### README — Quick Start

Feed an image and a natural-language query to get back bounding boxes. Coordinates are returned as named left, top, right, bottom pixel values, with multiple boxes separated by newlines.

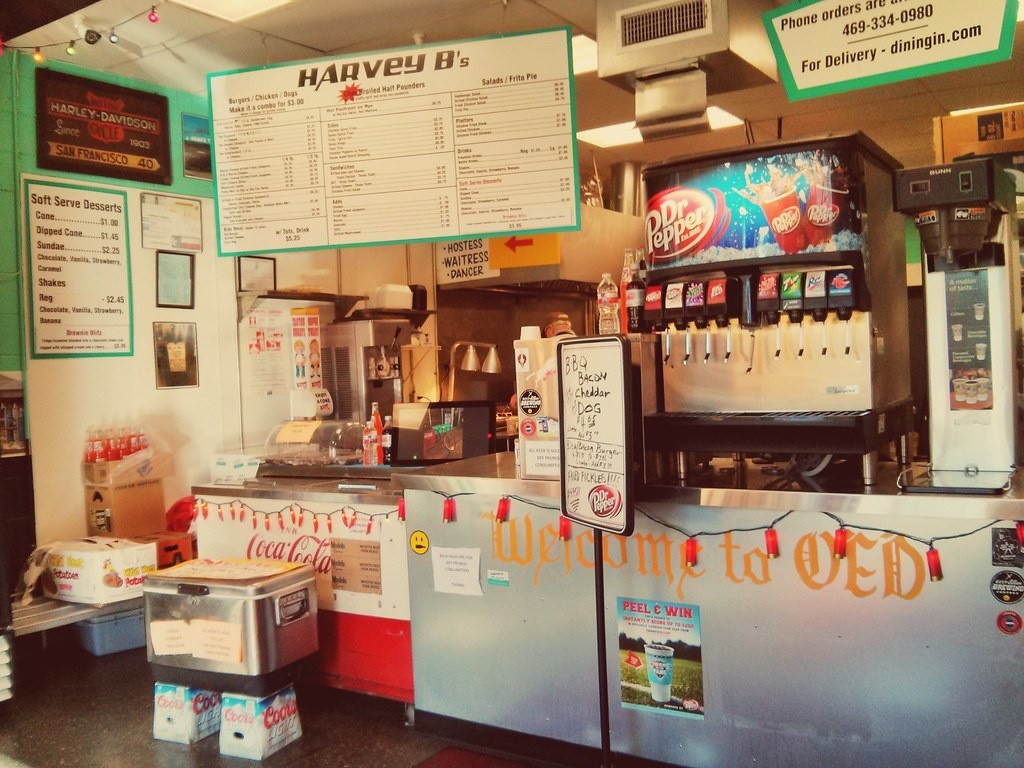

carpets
left=412, top=744, right=544, bottom=768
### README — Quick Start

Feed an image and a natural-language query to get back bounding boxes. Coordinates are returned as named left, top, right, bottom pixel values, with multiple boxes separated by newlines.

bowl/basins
left=520, top=327, right=540, bottom=340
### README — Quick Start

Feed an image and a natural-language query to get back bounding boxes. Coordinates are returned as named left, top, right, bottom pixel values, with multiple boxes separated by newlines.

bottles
left=362, top=420, right=378, bottom=466
left=381, top=416, right=396, bottom=465
left=84, top=427, right=148, bottom=462
left=370, top=402, right=384, bottom=465
left=618, top=245, right=647, bottom=332
left=597, top=273, right=620, bottom=335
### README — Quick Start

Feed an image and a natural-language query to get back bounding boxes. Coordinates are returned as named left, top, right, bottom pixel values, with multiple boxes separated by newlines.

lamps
left=447, top=339, right=504, bottom=401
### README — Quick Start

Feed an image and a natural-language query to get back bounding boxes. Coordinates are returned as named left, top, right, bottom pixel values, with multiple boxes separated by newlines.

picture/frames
left=155, top=250, right=196, bottom=310
left=238, top=254, right=277, bottom=293
left=152, top=321, right=200, bottom=390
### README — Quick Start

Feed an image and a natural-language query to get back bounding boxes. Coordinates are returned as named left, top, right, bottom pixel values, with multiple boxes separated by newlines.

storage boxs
left=941, top=103, right=1024, bottom=165
left=39, top=449, right=322, bottom=761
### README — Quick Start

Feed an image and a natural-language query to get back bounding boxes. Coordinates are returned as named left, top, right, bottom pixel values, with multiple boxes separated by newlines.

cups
left=644, top=644, right=674, bottom=703
left=952, top=324, right=963, bottom=341
left=976, top=343, right=987, bottom=361
left=952, top=379, right=966, bottom=401
left=964, top=380, right=978, bottom=404
left=976, top=377, right=989, bottom=401
left=760, top=186, right=805, bottom=255
left=974, top=302, right=985, bottom=320
left=804, top=184, right=848, bottom=246
